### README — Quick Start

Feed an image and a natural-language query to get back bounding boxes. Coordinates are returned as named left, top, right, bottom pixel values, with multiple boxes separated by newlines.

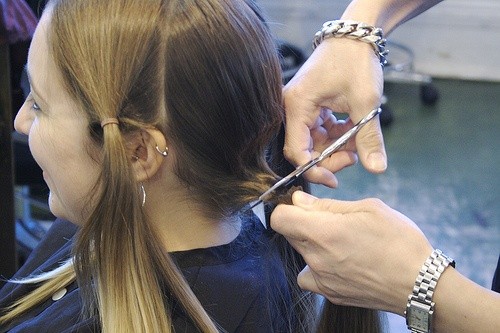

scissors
left=236, top=105, right=382, bottom=216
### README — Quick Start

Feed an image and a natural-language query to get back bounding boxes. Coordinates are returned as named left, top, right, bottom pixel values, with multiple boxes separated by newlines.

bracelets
left=311, top=20, right=389, bottom=66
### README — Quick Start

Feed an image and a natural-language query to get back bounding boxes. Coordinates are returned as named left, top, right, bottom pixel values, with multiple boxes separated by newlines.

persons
left=0, top=0, right=316, bottom=333
left=269, top=1, right=500, bottom=332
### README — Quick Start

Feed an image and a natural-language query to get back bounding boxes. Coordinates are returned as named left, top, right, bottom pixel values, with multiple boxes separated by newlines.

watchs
left=404, top=247, right=457, bottom=333
